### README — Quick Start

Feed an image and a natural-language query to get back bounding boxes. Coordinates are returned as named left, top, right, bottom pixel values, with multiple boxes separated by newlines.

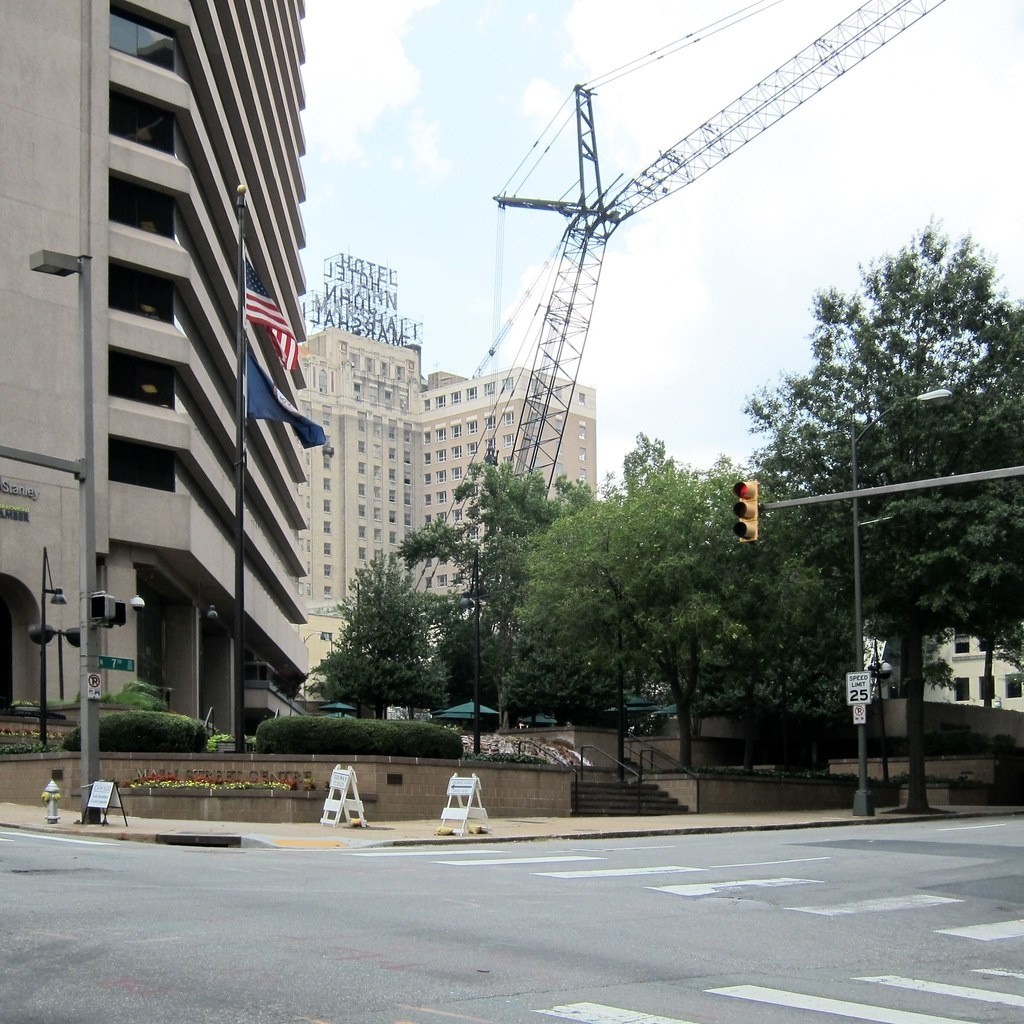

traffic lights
left=732, top=481, right=759, bottom=541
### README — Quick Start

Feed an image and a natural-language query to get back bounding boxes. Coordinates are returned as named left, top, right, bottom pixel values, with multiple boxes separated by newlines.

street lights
left=851, top=389, right=953, bottom=816
left=868, top=636, right=893, bottom=784
left=39, top=547, right=67, bottom=744
left=459, top=552, right=490, bottom=754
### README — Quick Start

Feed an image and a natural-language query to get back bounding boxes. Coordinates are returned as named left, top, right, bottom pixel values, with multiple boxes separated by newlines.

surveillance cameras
left=131, top=596, right=145, bottom=612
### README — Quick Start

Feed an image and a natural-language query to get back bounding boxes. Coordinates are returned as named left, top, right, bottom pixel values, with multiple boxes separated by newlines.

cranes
left=415, top=0, right=946, bottom=594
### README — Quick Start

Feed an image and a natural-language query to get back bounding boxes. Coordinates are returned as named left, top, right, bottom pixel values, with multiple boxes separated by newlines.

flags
left=247, top=352, right=327, bottom=449
left=242, top=239, right=299, bottom=372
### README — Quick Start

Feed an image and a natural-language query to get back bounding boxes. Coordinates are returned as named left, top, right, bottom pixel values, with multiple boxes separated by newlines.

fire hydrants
left=41, top=778, right=61, bottom=824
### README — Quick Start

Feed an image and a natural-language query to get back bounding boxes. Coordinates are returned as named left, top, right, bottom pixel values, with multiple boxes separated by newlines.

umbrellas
left=605, top=696, right=695, bottom=716
left=430, top=700, right=499, bottom=732
left=522, top=714, right=558, bottom=724
left=318, top=702, right=356, bottom=718
